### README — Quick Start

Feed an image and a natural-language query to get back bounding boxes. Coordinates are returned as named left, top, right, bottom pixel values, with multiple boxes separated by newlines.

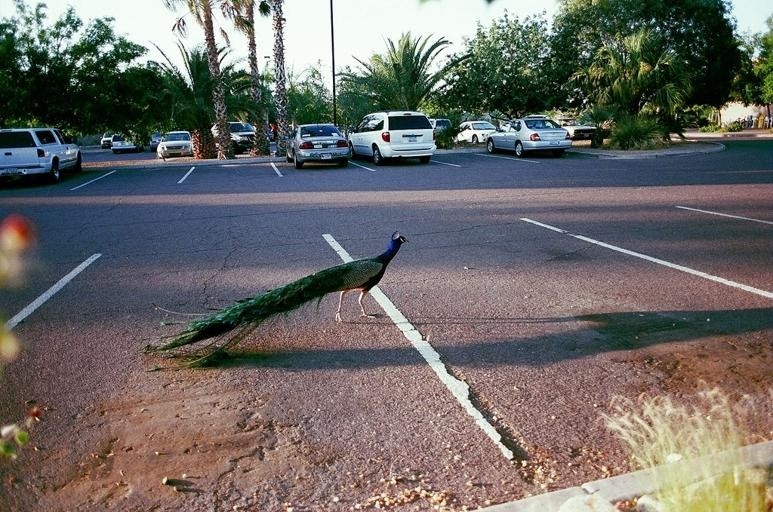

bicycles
left=732, top=116, right=749, bottom=131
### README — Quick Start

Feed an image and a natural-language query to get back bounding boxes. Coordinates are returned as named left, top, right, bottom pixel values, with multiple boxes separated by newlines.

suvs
left=211, top=121, right=256, bottom=155
left=428, top=118, right=453, bottom=138
left=347, top=110, right=437, bottom=166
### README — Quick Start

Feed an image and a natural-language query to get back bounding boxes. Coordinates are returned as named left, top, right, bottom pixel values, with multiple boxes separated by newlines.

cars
left=99, top=129, right=193, bottom=159
left=522, top=114, right=550, bottom=127
left=452, top=120, right=497, bottom=145
left=486, top=118, right=573, bottom=159
left=284, top=124, right=350, bottom=169
left=0, top=127, right=82, bottom=182
left=545, top=117, right=598, bottom=142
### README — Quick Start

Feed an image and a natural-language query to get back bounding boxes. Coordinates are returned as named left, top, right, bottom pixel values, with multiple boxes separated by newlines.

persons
left=270, top=122, right=278, bottom=139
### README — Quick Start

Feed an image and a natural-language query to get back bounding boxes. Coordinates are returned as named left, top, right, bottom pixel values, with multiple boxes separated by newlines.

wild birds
left=140, top=230, right=410, bottom=372
